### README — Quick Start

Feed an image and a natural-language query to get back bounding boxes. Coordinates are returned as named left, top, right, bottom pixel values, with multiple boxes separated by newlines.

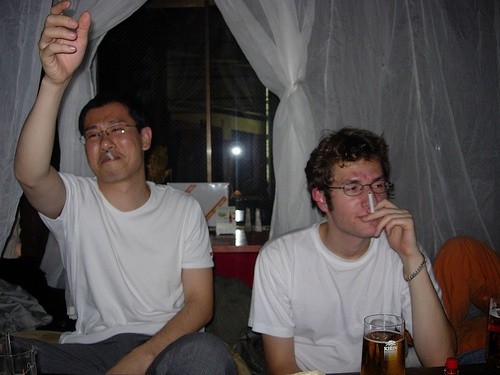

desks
left=211, top=230, right=269, bottom=287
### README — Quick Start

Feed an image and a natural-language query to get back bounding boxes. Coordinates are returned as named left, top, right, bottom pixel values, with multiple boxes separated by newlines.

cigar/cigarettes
left=368, top=191, right=374, bottom=214
left=105, top=149, right=115, bottom=161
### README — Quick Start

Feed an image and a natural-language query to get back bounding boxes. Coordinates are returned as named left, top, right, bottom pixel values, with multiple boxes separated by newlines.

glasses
left=78, top=123, right=135, bottom=143
left=328, top=182, right=386, bottom=197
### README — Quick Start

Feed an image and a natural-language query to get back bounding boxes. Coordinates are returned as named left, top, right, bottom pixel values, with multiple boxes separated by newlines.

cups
left=0, top=341, right=37, bottom=375
left=360, top=314, right=405, bottom=375
left=482, top=297, right=500, bottom=375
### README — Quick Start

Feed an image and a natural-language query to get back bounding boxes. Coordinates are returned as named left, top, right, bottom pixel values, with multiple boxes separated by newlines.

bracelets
left=405, top=253, right=427, bottom=283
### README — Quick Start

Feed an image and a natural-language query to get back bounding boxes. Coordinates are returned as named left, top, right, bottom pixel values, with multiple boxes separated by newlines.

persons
left=13, top=0, right=238, bottom=375
left=433, top=235, right=500, bottom=355
left=248, top=126, right=458, bottom=375
